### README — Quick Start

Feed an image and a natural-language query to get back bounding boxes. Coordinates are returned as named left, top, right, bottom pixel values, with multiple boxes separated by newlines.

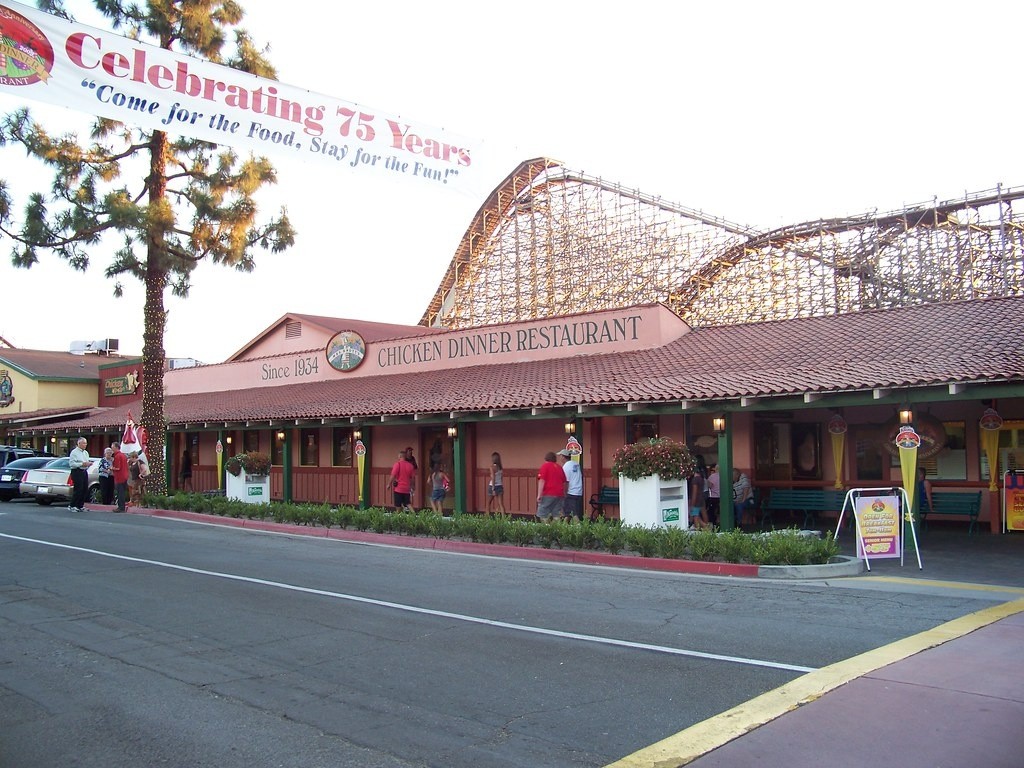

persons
left=426, top=461, right=450, bottom=518
left=917, top=467, right=935, bottom=513
left=67, top=437, right=93, bottom=512
left=688, top=455, right=754, bottom=531
left=387, top=447, right=418, bottom=514
left=98, top=442, right=147, bottom=513
left=485, top=452, right=505, bottom=520
left=537, top=449, right=582, bottom=526
left=180, top=450, right=195, bottom=494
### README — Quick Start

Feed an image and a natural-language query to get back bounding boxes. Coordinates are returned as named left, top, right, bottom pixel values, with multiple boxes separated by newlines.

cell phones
left=90, top=460, right=94, bottom=463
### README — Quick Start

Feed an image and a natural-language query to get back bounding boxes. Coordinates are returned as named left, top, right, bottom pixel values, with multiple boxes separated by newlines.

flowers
left=224, top=450, right=271, bottom=477
left=612, top=434, right=705, bottom=482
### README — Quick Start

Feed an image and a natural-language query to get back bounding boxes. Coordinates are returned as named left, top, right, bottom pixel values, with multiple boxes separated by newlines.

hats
left=555, top=449, right=572, bottom=456
left=405, top=446, right=413, bottom=451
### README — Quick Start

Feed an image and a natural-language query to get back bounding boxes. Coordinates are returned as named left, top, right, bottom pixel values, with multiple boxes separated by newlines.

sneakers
left=113, top=508, right=125, bottom=512
left=78, top=506, right=89, bottom=511
left=68, top=505, right=79, bottom=512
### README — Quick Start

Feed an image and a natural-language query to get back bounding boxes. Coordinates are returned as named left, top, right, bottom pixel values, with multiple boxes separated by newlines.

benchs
left=702, top=486, right=759, bottom=526
left=761, top=490, right=860, bottom=530
left=588, top=486, right=619, bottom=518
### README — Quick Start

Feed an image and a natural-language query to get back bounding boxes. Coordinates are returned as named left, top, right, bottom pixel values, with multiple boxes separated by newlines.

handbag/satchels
left=442, top=479, right=449, bottom=491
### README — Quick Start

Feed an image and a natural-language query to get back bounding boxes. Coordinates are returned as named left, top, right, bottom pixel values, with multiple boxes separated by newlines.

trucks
left=0, top=445, right=35, bottom=468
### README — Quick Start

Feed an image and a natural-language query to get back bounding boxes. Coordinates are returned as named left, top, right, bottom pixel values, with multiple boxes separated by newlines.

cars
left=20, top=456, right=105, bottom=507
left=0, top=457, right=67, bottom=503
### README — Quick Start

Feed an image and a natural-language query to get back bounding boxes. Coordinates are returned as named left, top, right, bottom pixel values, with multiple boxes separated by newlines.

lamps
left=227, top=434, right=232, bottom=445
left=277, top=431, right=286, bottom=441
left=899, top=408, right=912, bottom=426
left=565, top=421, right=575, bottom=436
left=447, top=425, right=458, bottom=439
left=51, top=436, right=56, bottom=444
left=880, top=491, right=983, bottom=537
left=353, top=429, right=361, bottom=439
left=714, top=418, right=725, bottom=432
